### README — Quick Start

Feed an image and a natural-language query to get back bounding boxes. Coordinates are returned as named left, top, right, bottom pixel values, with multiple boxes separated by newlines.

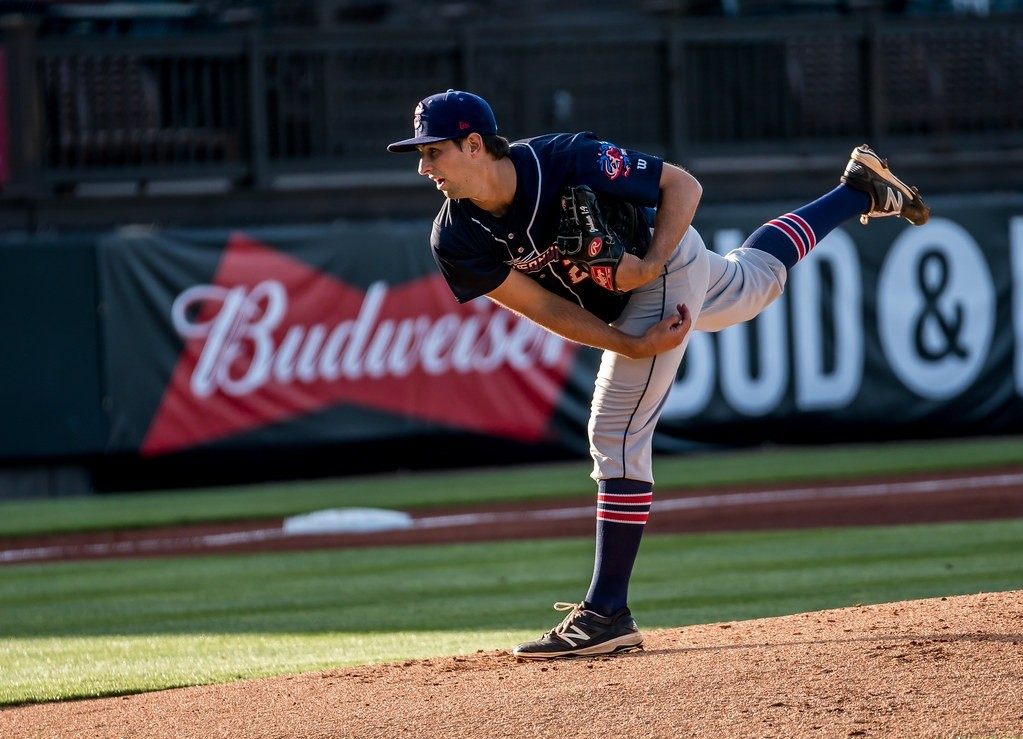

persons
left=387, top=87, right=930, bottom=661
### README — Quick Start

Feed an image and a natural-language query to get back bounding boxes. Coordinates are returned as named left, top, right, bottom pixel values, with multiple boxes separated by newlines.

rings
left=616, top=285, right=620, bottom=291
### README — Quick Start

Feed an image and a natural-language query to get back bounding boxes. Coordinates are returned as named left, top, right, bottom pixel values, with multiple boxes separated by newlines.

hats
left=387, top=88, right=496, bottom=153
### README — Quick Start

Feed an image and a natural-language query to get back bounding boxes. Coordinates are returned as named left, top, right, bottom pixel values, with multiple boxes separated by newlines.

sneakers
left=512, top=600, right=643, bottom=661
left=841, top=143, right=931, bottom=227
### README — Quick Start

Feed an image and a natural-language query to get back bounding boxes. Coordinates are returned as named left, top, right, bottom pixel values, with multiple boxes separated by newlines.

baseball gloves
left=554, top=182, right=639, bottom=297
